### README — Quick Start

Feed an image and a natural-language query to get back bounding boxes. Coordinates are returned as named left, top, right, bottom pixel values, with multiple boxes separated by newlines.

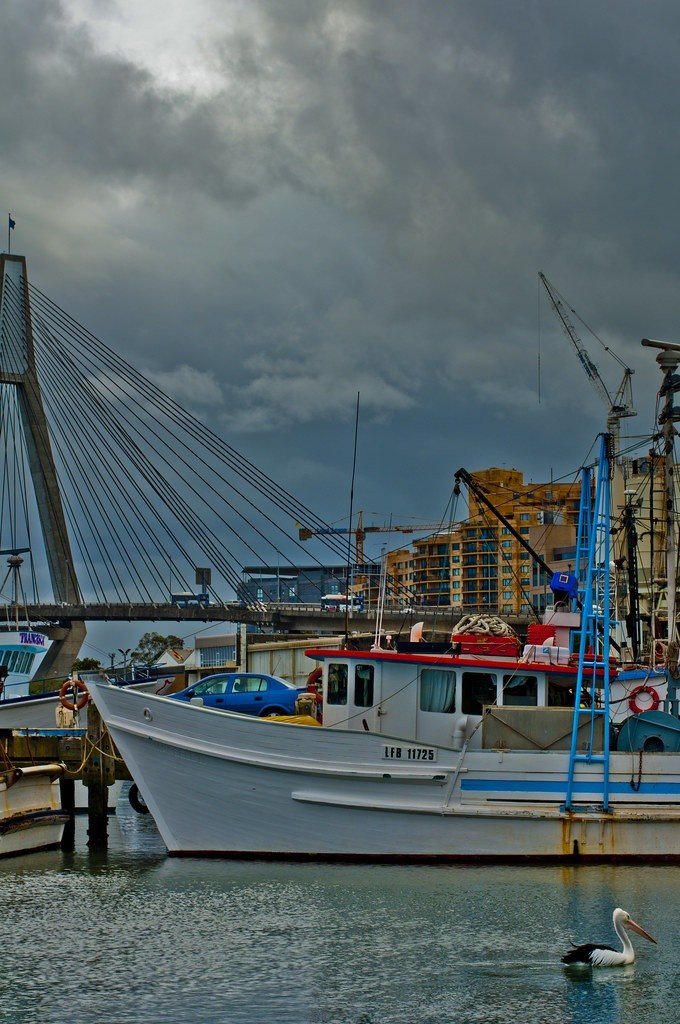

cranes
left=297, top=511, right=461, bottom=613
left=536, top=270, right=637, bottom=458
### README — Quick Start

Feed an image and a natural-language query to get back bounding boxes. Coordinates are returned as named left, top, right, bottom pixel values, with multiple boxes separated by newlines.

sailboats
left=80, top=259, right=680, bottom=855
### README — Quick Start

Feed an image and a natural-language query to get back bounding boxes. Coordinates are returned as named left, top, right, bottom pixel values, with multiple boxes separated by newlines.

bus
left=320, top=594, right=364, bottom=613
left=169, top=592, right=209, bottom=608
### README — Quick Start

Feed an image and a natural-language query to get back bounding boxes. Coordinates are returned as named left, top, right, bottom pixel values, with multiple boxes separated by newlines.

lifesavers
left=628, top=686, right=659, bottom=713
left=128, top=783, right=150, bottom=814
left=569, top=652, right=617, bottom=670
left=306, top=666, right=343, bottom=703
left=60, top=680, right=88, bottom=710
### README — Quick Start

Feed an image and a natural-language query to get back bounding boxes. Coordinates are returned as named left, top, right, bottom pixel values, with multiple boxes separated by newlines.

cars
left=55, top=601, right=71, bottom=608
left=161, top=673, right=310, bottom=717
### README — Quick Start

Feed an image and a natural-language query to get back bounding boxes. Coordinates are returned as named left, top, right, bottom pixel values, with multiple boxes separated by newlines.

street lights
left=118, top=647, right=132, bottom=683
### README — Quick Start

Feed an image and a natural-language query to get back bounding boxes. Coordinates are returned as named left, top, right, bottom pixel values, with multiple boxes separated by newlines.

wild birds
left=562, top=907, right=658, bottom=968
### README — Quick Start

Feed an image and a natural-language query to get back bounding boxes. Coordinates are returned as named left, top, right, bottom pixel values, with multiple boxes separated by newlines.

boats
left=0, top=664, right=175, bottom=815
left=0, top=759, right=70, bottom=857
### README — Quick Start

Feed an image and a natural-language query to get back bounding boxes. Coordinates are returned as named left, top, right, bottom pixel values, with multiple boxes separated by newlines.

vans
left=583, top=604, right=603, bottom=616
left=223, top=599, right=240, bottom=609
left=545, top=605, right=554, bottom=613
